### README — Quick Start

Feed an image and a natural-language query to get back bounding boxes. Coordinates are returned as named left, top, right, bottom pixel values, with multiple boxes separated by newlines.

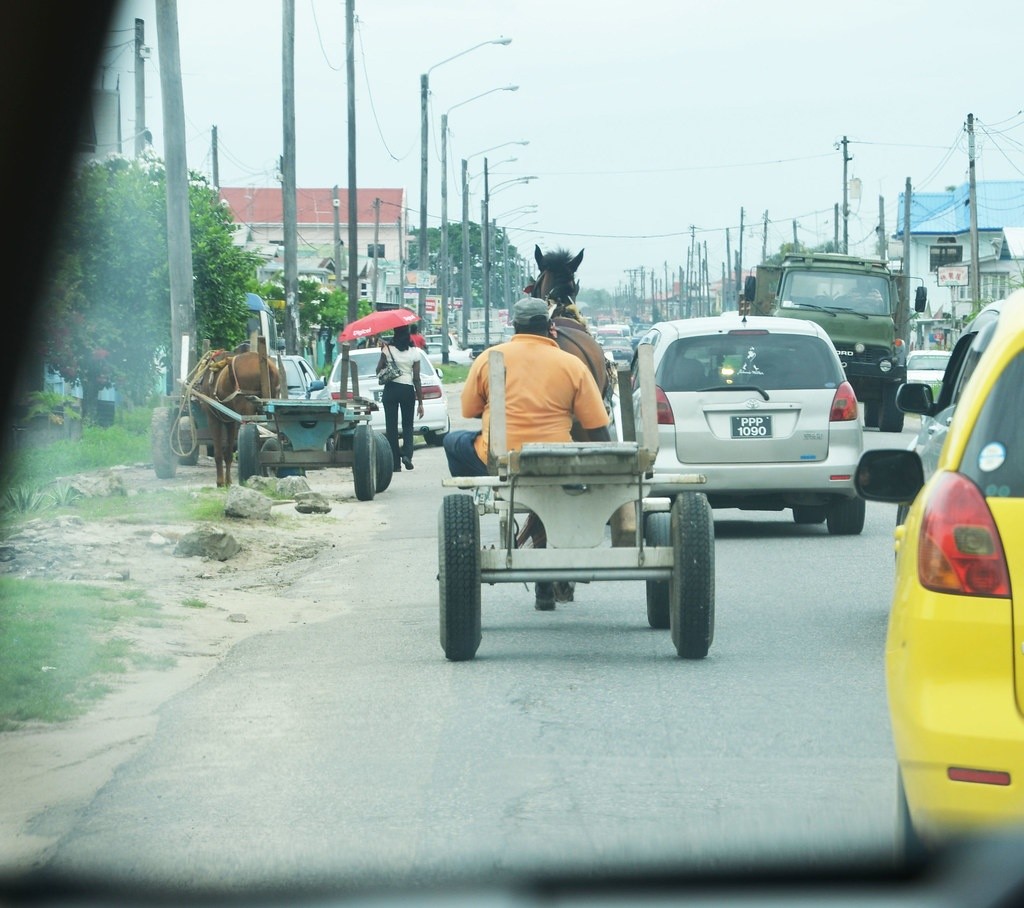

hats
left=508, top=296, right=550, bottom=326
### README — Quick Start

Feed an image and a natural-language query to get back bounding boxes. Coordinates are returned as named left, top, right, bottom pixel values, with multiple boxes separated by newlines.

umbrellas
left=339, top=307, right=421, bottom=347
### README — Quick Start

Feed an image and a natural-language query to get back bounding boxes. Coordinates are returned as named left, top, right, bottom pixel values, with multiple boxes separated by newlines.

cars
left=623, top=313, right=868, bottom=536
left=902, top=350, right=956, bottom=405
left=267, top=354, right=324, bottom=405
left=451, top=306, right=658, bottom=372
left=852, top=287, right=1024, bottom=877
left=887, top=299, right=1005, bottom=534
left=326, top=346, right=452, bottom=448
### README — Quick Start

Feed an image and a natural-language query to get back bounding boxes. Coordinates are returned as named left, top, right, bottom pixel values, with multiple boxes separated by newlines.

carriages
left=172, top=339, right=394, bottom=503
left=436, top=243, right=722, bottom=664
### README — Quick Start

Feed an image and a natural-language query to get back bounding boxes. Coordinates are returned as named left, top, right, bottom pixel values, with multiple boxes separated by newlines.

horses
left=196, top=353, right=279, bottom=488
left=517, top=244, right=615, bottom=610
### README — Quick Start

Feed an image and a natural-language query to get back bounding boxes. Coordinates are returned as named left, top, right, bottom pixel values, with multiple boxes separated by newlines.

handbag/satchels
left=378, top=342, right=402, bottom=385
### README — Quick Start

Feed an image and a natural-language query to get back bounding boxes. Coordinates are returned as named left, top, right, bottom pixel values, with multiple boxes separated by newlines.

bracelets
left=418, top=400, right=422, bottom=406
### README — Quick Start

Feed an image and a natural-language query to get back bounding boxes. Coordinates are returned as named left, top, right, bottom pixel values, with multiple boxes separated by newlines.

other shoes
left=393, top=466, right=402, bottom=472
left=402, top=456, right=414, bottom=470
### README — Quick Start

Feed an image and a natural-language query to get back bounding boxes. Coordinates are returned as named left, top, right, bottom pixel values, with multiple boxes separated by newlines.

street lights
left=439, top=84, right=519, bottom=362
left=417, top=36, right=513, bottom=360
left=460, top=139, right=530, bottom=347
left=482, top=157, right=541, bottom=342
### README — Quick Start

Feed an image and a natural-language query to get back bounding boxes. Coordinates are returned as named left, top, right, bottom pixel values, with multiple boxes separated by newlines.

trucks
left=741, top=256, right=929, bottom=431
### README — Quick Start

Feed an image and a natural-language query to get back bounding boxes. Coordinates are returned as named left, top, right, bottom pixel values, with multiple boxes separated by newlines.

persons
left=408, top=325, right=429, bottom=355
left=443, top=298, right=613, bottom=492
left=377, top=325, right=425, bottom=471
